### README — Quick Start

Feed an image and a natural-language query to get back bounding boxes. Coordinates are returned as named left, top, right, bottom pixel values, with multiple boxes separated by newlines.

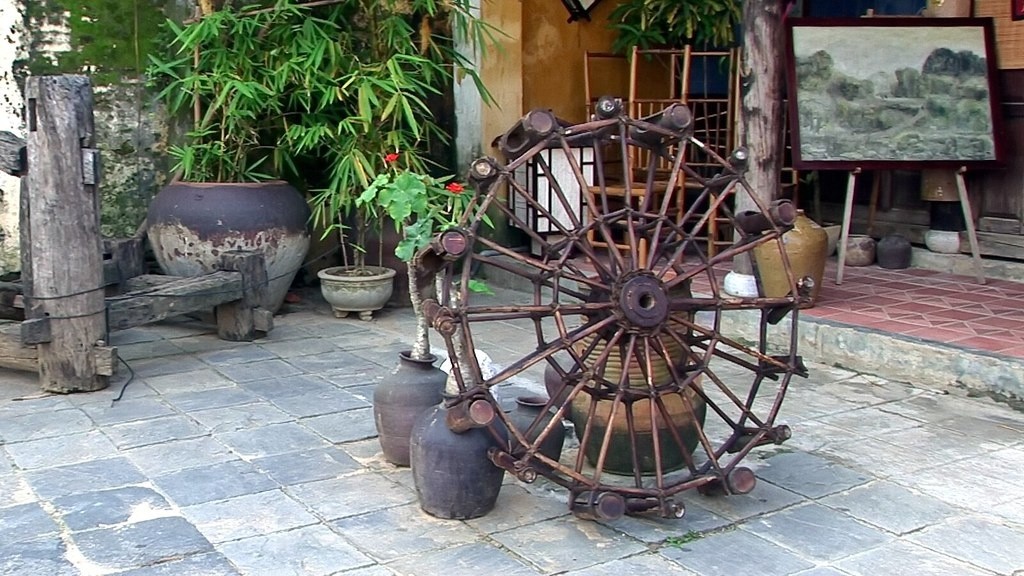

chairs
left=584, top=44, right=799, bottom=269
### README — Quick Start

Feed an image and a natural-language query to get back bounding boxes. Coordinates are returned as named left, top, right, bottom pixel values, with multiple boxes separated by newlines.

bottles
left=506, top=270, right=707, bottom=476
left=753, top=209, right=829, bottom=309
left=925, top=230, right=960, bottom=254
left=835, top=234, right=875, bottom=267
left=876, top=232, right=912, bottom=269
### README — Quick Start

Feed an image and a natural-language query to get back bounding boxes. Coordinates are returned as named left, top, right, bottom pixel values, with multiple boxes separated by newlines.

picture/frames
left=784, top=16, right=1005, bottom=170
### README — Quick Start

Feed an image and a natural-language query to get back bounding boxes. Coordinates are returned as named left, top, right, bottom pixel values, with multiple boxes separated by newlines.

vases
left=374, top=351, right=446, bottom=467
left=409, top=393, right=506, bottom=520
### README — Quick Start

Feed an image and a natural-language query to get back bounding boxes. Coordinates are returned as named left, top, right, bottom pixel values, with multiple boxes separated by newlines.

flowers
left=353, top=152, right=496, bottom=395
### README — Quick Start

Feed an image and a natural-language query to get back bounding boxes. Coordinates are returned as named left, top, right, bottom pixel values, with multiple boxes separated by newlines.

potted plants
left=318, top=206, right=404, bottom=309
left=144, top=0, right=511, bottom=321
